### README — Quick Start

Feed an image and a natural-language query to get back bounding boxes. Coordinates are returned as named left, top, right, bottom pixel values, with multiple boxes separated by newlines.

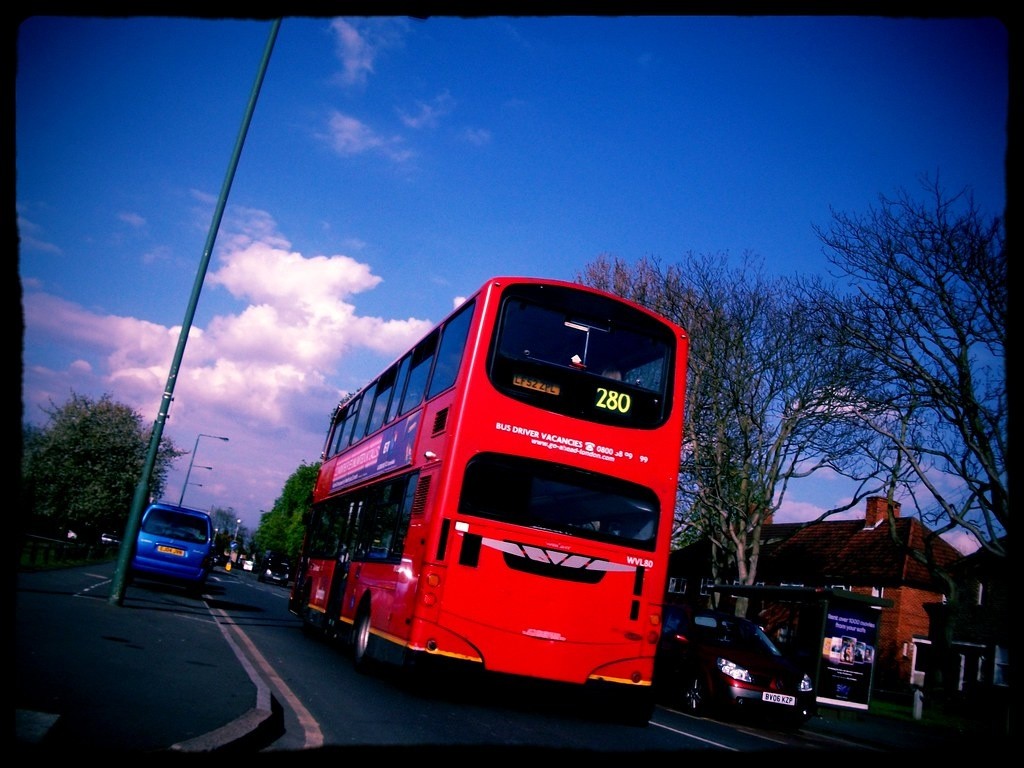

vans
left=131, top=502, right=214, bottom=598
left=257, top=551, right=292, bottom=586
left=243, top=560, right=254, bottom=573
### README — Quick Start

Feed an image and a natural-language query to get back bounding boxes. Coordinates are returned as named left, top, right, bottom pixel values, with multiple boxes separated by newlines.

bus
left=288, top=280, right=691, bottom=701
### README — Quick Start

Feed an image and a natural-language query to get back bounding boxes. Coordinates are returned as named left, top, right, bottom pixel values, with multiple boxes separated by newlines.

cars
left=651, top=602, right=815, bottom=732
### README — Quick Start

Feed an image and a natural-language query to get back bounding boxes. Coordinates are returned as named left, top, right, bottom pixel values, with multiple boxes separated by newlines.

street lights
left=178, top=433, right=229, bottom=506
